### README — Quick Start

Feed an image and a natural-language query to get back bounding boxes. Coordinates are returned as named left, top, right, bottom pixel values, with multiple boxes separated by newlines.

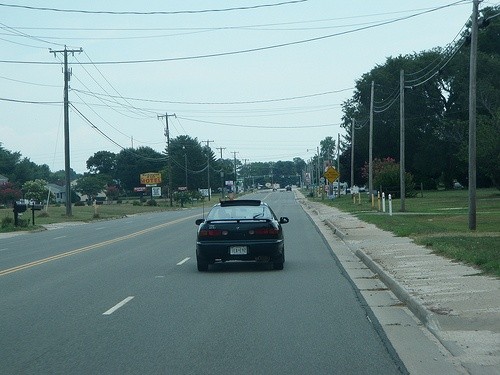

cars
left=285, top=187, right=292, bottom=191
left=273, top=187, right=277, bottom=191
left=195, top=199, right=289, bottom=273
